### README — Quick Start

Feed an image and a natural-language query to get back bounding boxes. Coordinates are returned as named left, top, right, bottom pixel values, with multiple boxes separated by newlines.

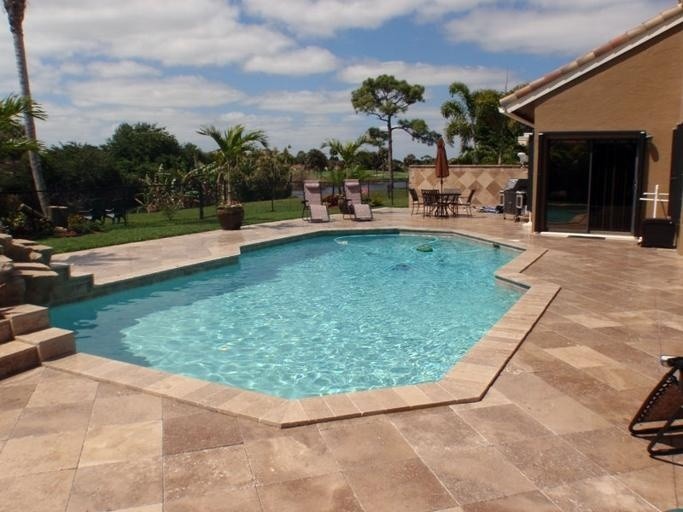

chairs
left=104, top=199, right=130, bottom=225
left=407, top=186, right=476, bottom=219
left=77, top=197, right=109, bottom=226
left=341, top=178, right=372, bottom=221
left=627, top=354, right=683, bottom=466
left=299, top=180, right=329, bottom=223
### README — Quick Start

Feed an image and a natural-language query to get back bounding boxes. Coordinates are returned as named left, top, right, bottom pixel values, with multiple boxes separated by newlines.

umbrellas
left=436, top=138, right=449, bottom=215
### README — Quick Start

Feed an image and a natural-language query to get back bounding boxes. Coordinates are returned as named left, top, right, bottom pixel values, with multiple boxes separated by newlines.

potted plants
left=196, top=125, right=270, bottom=230
left=320, top=135, right=382, bottom=211
